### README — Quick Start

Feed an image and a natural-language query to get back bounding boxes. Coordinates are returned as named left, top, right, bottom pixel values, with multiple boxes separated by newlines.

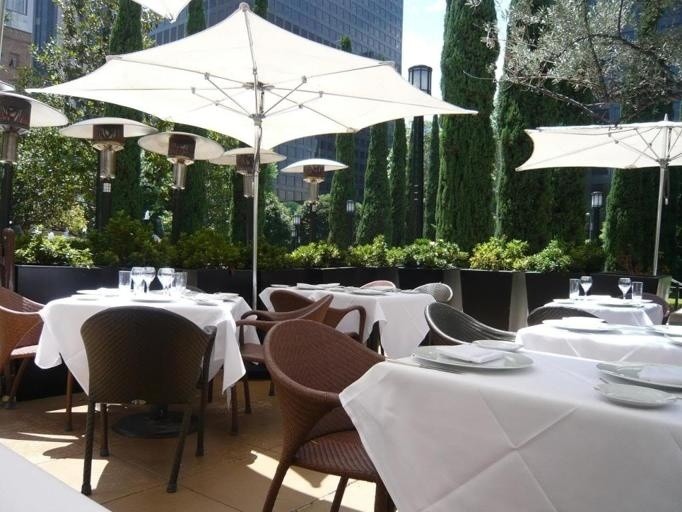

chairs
left=618, top=293, right=671, bottom=325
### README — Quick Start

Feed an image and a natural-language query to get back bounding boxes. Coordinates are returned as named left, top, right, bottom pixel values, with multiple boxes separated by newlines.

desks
left=375, top=296, right=682, bottom=512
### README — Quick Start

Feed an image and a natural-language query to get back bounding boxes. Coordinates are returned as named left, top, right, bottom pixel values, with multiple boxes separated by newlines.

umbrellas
left=24, top=1, right=478, bottom=312
left=515, top=113, right=682, bottom=277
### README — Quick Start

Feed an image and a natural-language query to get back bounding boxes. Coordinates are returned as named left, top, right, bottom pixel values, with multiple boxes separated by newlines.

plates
left=349, top=289, right=385, bottom=294
left=73, top=290, right=239, bottom=303
left=413, top=317, right=682, bottom=408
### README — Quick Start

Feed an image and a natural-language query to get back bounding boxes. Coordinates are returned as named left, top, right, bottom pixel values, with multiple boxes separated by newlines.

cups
left=173, top=272, right=187, bottom=291
left=632, top=282, right=642, bottom=299
left=569, top=279, right=579, bottom=300
left=119, top=271, right=131, bottom=287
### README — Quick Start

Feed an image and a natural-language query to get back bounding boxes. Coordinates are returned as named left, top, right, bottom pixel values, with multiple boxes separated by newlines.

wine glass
left=131, top=266, right=176, bottom=294
left=580, top=276, right=592, bottom=299
left=618, top=278, right=631, bottom=299
